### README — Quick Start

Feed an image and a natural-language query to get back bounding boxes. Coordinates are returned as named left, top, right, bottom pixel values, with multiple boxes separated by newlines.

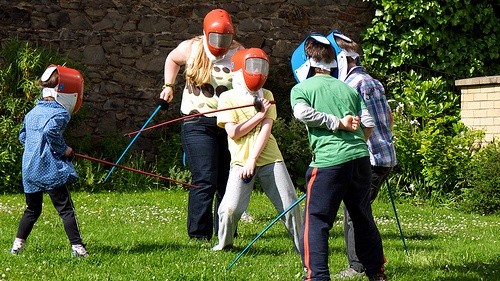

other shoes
left=210, top=244, right=234, bottom=251
left=71, top=244, right=90, bottom=258
left=335, top=268, right=366, bottom=280
left=368, top=273, right=388, bottom=281
left=10, top=237, right=26, bottom=255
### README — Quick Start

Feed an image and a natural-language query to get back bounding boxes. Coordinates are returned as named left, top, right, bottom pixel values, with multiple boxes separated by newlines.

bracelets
left=162, top=84, right=175, bottom=91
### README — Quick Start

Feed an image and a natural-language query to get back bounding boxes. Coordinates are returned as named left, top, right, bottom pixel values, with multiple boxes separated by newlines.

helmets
left=291, top=30, right=360, bottom=83
left=42, top=64, right=84, bottom=115
left=230, top=48, right=270, bottom=92
left=203, top=9, right=235, bottom=59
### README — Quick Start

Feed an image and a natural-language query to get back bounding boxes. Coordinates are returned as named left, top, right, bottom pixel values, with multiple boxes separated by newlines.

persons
left=290, top=34, right=385, bottom=281
left=212, top=48, right=303, bottom=253
left=326, top=30, right=397, bottom=278
left=160, top=9, right=246, bottom=240
left=11, top=64, right=91, bottom=257
